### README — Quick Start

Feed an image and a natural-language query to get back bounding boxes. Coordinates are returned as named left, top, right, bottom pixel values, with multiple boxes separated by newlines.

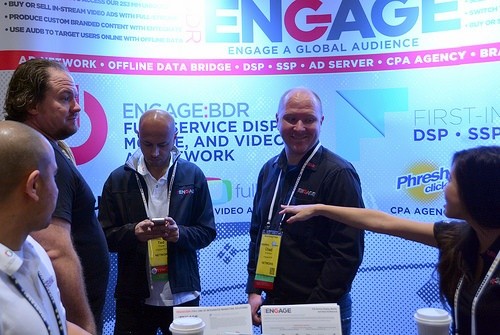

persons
left=1, top=118, right=92, bottom=335
left=100, top=109, right=217, bottom=334
left=279, top=146, right=496, bottom=334
left=242, top=88, right=365, bottom=335
left=1, top=58, right=110, bottom=335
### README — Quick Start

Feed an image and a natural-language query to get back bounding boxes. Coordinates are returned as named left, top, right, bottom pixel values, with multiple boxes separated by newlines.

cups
left=413, top=308, right=453, bottom=335
left=169, top=316, right=206, bottom=335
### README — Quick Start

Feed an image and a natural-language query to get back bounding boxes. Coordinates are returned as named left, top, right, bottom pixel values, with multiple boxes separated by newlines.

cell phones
left=152, top=218, right=165, bottom=226
